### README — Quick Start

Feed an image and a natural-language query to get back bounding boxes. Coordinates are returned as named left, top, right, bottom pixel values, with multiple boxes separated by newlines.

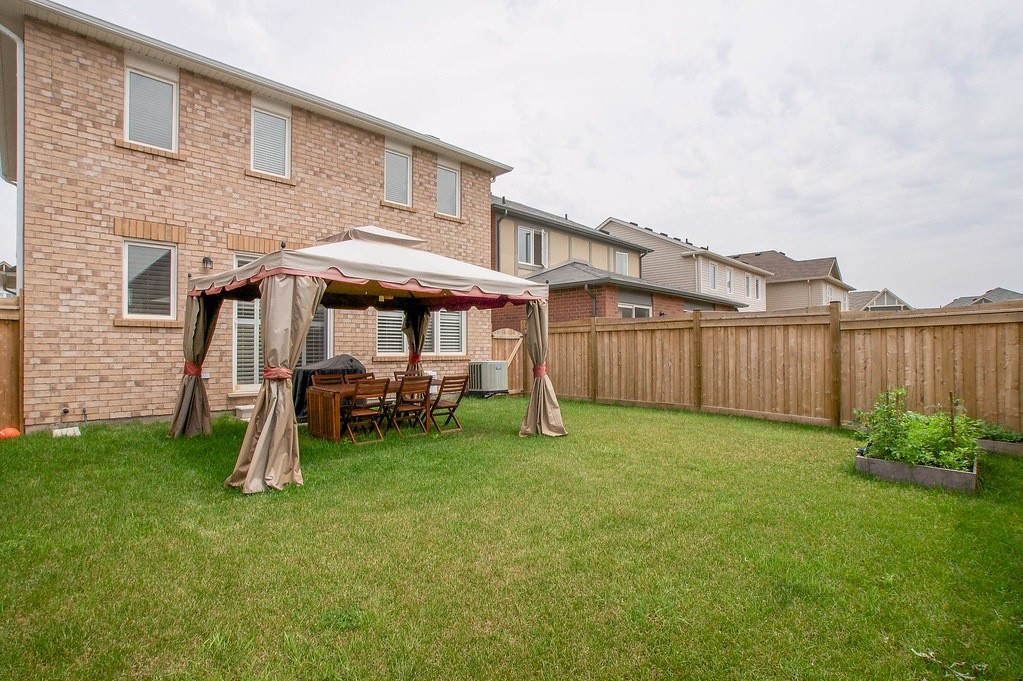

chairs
left=312, top=371, right=470, bottom=447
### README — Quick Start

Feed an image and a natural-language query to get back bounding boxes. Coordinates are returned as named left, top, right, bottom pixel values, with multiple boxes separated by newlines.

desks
left=306, top=380, right=443, bottom=444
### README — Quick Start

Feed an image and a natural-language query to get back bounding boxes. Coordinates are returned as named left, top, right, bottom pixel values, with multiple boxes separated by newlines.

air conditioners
left=468, top=360, right=509, bottom=392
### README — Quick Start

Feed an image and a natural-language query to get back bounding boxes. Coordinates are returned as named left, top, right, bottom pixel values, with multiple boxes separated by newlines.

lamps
left=203, top=257, right=214, bottom=269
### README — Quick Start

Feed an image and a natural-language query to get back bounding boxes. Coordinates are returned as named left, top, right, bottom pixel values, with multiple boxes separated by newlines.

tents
left=165, top=223, right=569, bottom=494
